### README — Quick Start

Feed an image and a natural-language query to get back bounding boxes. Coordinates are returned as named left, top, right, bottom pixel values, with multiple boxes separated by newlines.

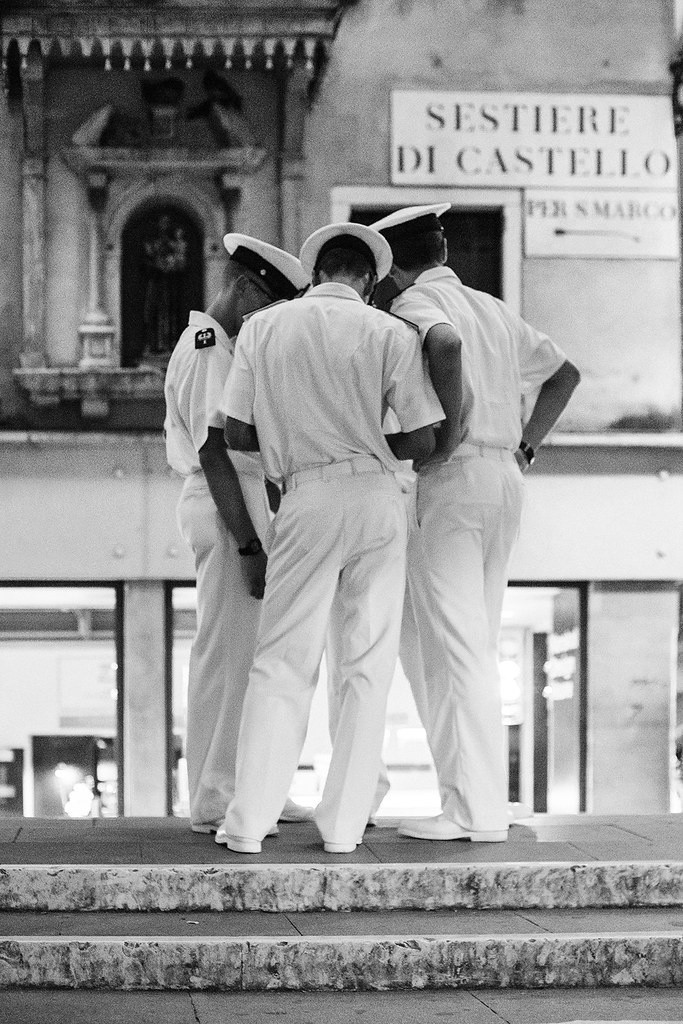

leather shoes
left=215, top=822, right=261, bottom=853
left=279, top=798, right=314, bottom=822
left=325, top=838, right=362, bottom=853
left=191, top=814, right=278, bottom=835
left=398, top=811, right=508, bottom=844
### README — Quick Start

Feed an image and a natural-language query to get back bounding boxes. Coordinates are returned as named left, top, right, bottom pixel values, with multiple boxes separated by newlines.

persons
left=371, top=201, right=581, bottom=844
left=165, top=227, right=307, bottom=836
left=214, top=220, right=446, bottom=858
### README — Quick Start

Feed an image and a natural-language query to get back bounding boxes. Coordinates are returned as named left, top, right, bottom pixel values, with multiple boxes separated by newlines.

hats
left=298, top=222, right=393, bottom=285
left=367, top=203, right=451, bottom=243
left=222, top=233, right=312, bottom=300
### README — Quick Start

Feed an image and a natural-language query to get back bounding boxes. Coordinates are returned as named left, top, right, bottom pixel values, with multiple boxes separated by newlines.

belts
left=182, top=473, right=210, bottom=490
left=454, top=443, right=517, bottom=462
left=288, top=457, right=382, bottom=489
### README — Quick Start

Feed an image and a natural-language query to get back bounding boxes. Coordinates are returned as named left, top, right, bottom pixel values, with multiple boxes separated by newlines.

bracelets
left=519, top=441, right=535, bottom=465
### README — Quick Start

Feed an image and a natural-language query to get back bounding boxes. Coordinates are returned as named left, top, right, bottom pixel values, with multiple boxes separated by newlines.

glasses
left=249, top=279, right=276, bottom=304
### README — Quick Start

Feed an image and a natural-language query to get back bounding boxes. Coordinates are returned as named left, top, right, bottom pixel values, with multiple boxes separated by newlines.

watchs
left=238, top=538, right=262, bottom=557
